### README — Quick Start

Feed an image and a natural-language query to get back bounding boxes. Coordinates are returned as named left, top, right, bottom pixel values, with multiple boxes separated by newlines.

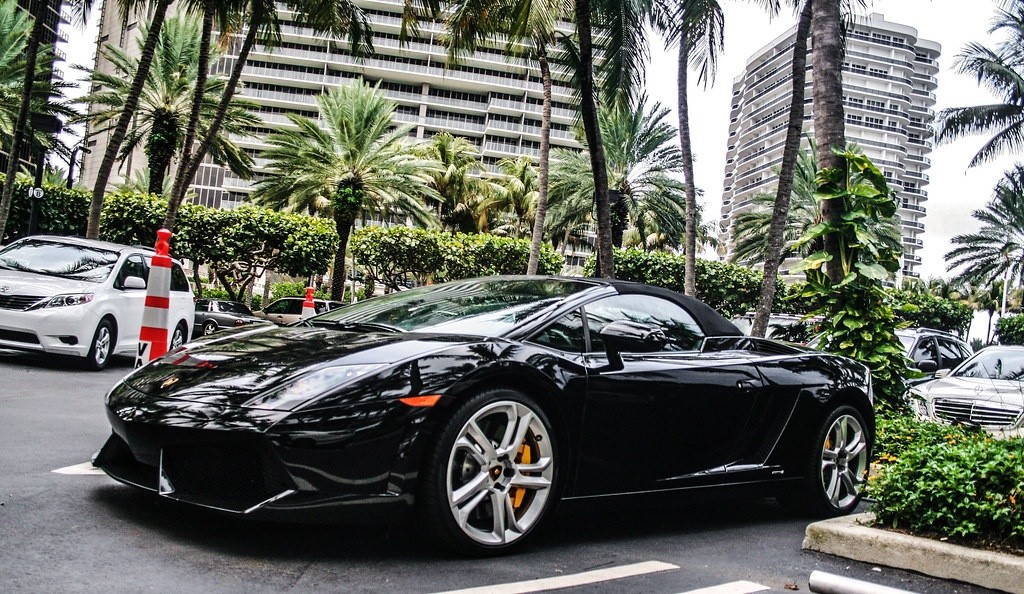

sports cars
left=91, top=275, right=876, bottom=559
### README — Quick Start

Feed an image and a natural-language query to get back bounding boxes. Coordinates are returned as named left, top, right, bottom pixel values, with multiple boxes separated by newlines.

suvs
left=250, top=295, right=347, bottom=328
left=804, top=327, right=976, bottom=388
left=732, top=313, right=834, bottom=346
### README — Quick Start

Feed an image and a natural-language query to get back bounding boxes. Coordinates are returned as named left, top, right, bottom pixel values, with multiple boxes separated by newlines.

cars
left=902, top=345, right=1024, bottom=441
left=0, top=235, right=198, bottom=370
left=192, top=297, right=275, bottom=338
